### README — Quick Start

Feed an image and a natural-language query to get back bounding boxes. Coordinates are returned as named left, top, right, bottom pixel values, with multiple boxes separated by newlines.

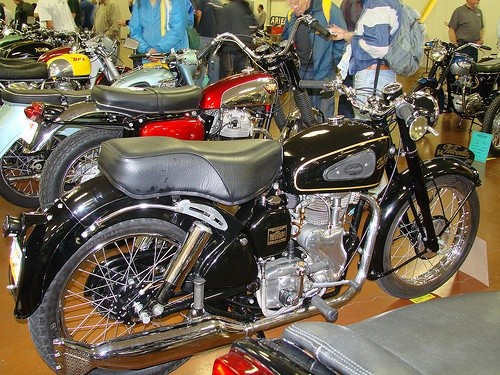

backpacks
left=384, top=0, right=426, bottom=78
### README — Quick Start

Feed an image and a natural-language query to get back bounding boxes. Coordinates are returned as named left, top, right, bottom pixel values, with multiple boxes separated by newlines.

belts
left=366, top=62, right=390, bottom=70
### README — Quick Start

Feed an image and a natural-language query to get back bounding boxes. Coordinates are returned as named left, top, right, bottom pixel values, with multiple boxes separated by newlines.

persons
left=338, top=0, right=363, bottom=121
left=0, top=0, right=267, bottom=87
left=129, top=0, right=194, bottom=69
left=282, top=0, right=347, bottom=121
left=447, top=0, right=485, bottom=63
left=323, top=0, right=403, bottom=127
left=90, top=0, right=121, bottom=66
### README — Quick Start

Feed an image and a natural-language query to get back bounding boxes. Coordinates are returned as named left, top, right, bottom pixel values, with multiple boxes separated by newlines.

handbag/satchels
left=187, top=26, right=201, bottom=50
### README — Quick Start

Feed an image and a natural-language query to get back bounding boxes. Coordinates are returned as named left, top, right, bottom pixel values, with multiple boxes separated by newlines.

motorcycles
left=411, top=38, right=500, bottom=135
left=1, top=26, right=119, bottom=85
left=481, top=95, right=500, bottom=158
left=1, top=78, right=481, bottom=375
left=210, top=288, right=500, bottom=375
left=37, top=15, right=330, bottom=207
left=1, top=48, right=208, bottom=207
